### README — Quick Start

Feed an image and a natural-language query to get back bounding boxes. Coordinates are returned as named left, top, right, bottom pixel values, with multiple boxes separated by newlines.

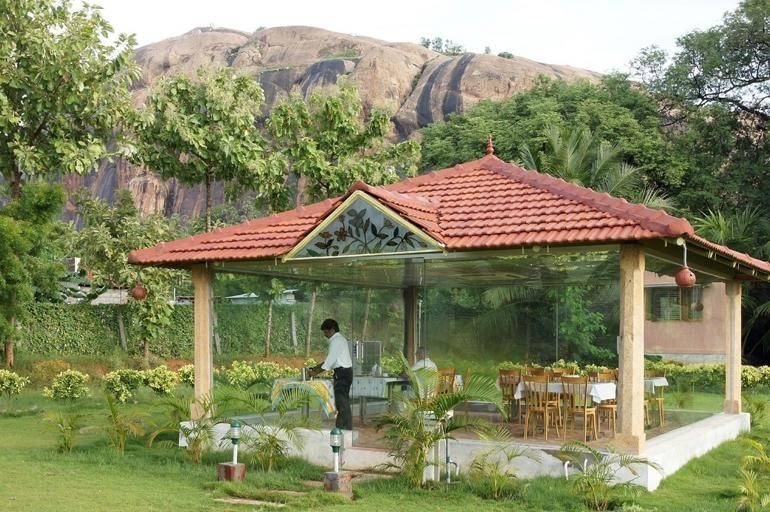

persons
left=308, top=318, right=354, bottom=430
left=394, top=346, right=438, bottom=418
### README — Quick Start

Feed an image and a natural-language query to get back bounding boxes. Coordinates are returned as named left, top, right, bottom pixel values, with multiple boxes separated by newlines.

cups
left=383, top=373, right=389, bottom=378
left=301, top=367, right=314, bottom=381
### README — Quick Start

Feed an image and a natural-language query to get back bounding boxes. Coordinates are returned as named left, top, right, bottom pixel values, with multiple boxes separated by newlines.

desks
left=273, top=375, right=408, bottom=425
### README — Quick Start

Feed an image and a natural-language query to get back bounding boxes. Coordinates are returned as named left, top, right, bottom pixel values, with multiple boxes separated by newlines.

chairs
left=499, top=366, right=668, bottom=444
left=409, top=367, right=471, bottom=432
